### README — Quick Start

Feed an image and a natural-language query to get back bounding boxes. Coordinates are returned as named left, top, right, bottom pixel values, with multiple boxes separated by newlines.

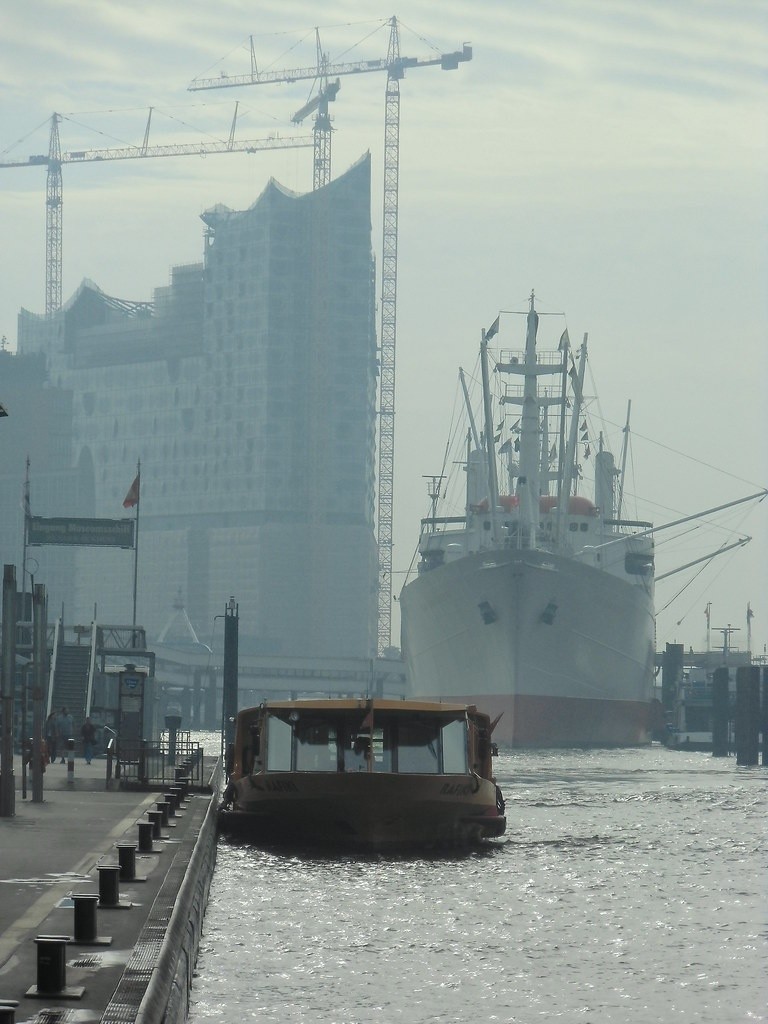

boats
left=216, top=684, right=509, bottom=846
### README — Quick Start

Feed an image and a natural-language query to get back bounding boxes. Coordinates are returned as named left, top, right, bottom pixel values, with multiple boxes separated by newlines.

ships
left=395, top=288, right=768, bottom=749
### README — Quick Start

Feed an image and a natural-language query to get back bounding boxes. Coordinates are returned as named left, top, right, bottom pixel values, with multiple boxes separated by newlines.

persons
left=45, top=705, right=97, bottom=765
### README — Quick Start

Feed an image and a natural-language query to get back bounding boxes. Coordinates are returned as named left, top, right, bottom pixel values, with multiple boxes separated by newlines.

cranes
left=183, top=13, right=474, bottom=659
left=1, top=99, right=321, bottom=389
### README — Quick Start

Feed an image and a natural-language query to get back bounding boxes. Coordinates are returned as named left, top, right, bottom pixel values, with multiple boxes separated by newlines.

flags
left=122, top=474, right=139, bottom=508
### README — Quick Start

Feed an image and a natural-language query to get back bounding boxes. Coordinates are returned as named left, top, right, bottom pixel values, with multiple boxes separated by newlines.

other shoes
left=87, top=761, right=90, bottom=764
left=59, top=759, right=65, bottom=764
left=51, top=760, right=54, bottom=763
